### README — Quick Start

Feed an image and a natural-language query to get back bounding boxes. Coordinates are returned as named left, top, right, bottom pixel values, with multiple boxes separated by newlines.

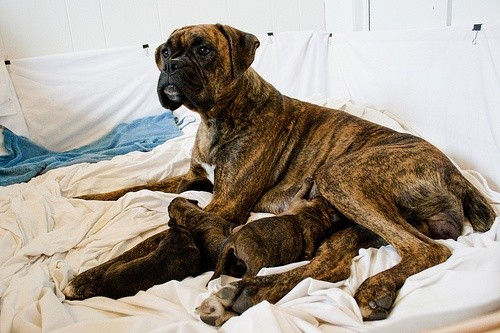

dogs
left=205, top=195, right=343, bottom=287
left=101, top=197, right=247, bottom=299
left=63, top=24, right=495, bottom=328
